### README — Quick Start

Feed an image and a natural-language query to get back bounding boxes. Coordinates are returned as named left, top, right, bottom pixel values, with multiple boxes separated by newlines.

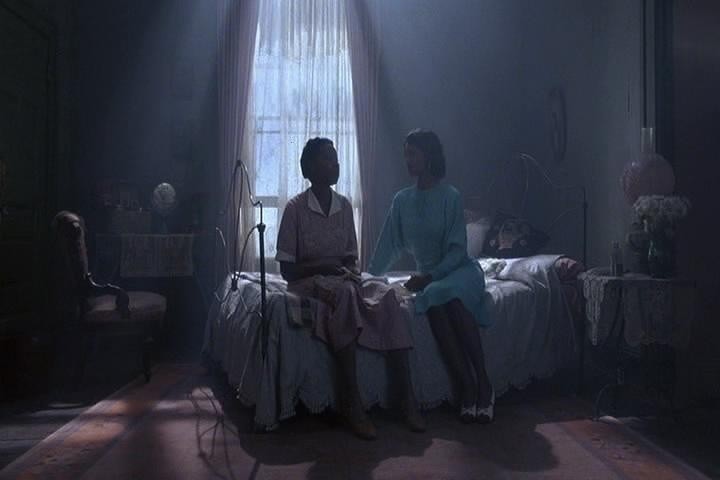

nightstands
left=88, top=232, right=194, bottom=369
left=575, top=266, right=694, bottom=425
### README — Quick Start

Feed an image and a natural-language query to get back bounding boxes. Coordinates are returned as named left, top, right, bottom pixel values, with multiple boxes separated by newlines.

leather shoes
left=477, top=381, right=495, bottom=422
left=459, top=402, right=476, bottom=421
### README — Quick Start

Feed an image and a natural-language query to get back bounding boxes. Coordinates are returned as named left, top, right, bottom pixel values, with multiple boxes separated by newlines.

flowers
left=633, top=193, right=692, bottom=234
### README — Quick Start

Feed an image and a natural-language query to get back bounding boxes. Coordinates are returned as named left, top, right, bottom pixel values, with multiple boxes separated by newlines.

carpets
left=0, top=357, right=713, bottom=480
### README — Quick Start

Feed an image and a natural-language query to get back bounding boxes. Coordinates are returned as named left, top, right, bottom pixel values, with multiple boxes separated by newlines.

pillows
left=479, top=209, right=550, bottom=258
left=465, top=216, right=491, bottom=258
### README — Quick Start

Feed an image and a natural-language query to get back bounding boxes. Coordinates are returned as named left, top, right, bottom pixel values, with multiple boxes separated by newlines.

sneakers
left=393, top=404, right=427, bottom=433
left=351, top=420, right=377, bottom=439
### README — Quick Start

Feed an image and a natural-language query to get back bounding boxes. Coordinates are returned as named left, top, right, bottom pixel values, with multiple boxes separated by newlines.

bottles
left=647, top=238, right=660, bottom=276
left=609, top=240, right=624, bottom=276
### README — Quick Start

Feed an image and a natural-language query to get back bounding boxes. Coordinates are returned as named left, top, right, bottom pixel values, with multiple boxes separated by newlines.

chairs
left=48, top=209, right=168, bottom=383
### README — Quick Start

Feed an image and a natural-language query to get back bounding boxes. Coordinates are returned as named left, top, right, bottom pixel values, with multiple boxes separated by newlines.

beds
left=202, top=160, right=587, bottom=434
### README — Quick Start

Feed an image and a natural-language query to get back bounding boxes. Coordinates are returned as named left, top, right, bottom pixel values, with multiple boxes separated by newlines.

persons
left=366, top=127, right=500, bottom=424
left=276, top=138, right=431, bottom=440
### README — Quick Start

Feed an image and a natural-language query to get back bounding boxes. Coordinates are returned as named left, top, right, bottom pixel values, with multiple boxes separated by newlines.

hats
left=618, top=151, right=691, bottom=223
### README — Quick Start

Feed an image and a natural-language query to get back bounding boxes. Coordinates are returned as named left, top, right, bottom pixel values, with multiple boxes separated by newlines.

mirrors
left=144, top=178, right=184, bottom=234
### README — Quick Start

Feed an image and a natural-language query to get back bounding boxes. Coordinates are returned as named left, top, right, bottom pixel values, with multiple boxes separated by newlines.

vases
left=646, top=230, right=674, bottom=279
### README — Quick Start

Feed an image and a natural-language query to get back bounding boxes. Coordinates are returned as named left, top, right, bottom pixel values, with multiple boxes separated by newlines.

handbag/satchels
left=481, top=217, right=550, bottom=259
